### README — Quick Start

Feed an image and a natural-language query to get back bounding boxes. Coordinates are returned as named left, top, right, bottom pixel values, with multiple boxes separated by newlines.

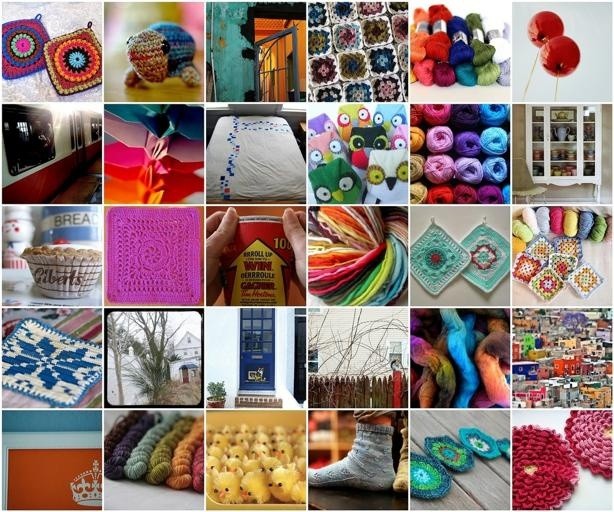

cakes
left=21, top=244, right=102, bottom=300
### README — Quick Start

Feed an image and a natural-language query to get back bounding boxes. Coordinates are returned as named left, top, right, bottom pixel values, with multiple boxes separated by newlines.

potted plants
left=205, top=381, right=227, bottom=408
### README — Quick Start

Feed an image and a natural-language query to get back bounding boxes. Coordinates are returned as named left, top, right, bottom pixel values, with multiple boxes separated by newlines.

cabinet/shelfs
left=523, top=105, right=602, bottom=203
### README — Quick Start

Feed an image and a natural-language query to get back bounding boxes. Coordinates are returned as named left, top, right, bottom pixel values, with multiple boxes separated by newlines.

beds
left=205, top=116, right=305, bottom=203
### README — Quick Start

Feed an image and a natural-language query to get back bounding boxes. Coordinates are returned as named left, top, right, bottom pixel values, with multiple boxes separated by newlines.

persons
left=206, top=207, right=306, bottom=305
left=309, top=411, right=408, bottom=492
